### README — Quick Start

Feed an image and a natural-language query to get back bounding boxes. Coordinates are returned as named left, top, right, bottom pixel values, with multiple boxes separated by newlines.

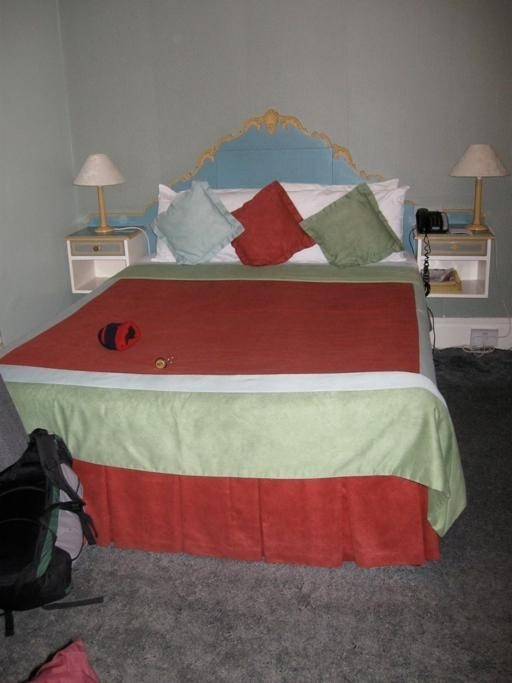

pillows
left=148, top=173, right=414, bottom=270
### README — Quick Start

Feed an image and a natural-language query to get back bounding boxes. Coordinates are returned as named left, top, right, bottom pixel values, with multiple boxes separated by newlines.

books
left=420, top=266, right=462, bottom=293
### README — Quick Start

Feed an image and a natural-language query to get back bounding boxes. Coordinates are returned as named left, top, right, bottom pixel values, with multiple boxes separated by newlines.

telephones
left=416, top=208, right=449, bottom=234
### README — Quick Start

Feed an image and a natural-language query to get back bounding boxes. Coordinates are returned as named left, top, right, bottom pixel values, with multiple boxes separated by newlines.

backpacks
left=0, top=428, right=96, bottom=632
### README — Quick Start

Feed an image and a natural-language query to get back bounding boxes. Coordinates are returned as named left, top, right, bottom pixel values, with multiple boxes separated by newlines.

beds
left=0, top=107, right=463, bottom=566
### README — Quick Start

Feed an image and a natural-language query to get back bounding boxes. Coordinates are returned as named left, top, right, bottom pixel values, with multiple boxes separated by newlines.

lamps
left=72, top=152, right=125, bottom=233
left=448, top=144, right=509, bottom=232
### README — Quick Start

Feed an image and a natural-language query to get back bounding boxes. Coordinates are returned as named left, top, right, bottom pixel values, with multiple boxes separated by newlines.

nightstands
left=65, top=225, right=150, bottom=294
left=413, top=224, right=497, bottom=300
